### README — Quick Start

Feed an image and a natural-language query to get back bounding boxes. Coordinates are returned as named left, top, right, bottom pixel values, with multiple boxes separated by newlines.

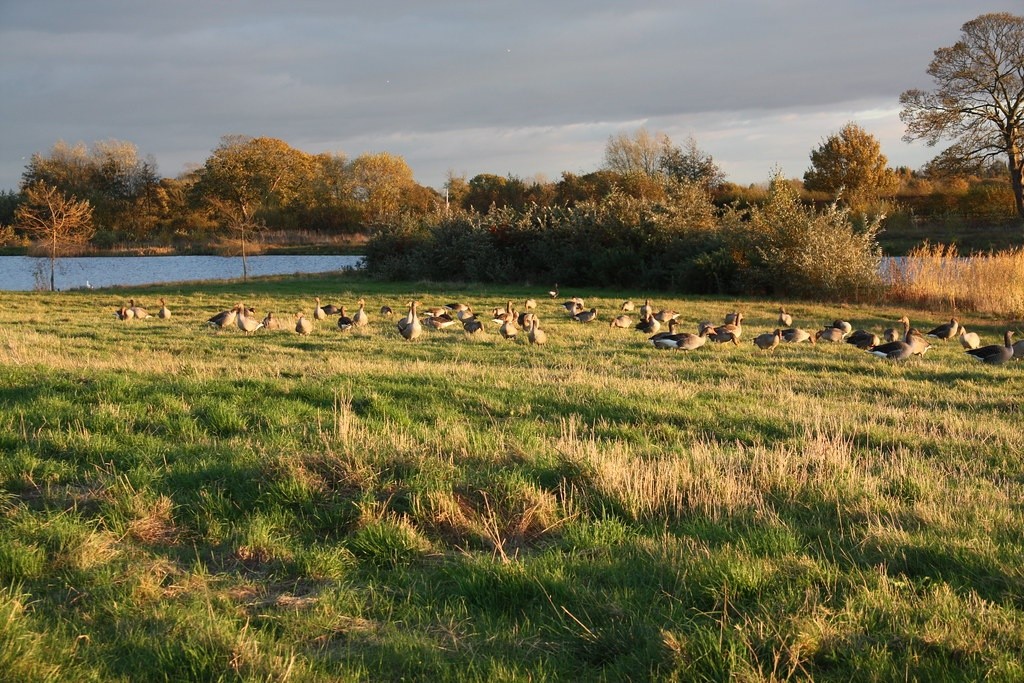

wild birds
left=560, top=296, right=635, bottom=330
left=816, top=314, right=938, bottom=365
left=750, top=305, right=816, bottom=354
left=204, top=303, right=274, bottom=337
left=86, top=280, right=94, bottom=290
left=395, top=299, right=486, bottom=344
left=110, top=297, right=172, bottom=323
left=547, top=281, right=560, bottom=300
left=490, top=298, right=548, bottom=346
left=380, top=305, right=393, bottom=318
left=292, top=310, right=315, bottom=342
left=925, top=316, right=1024, bottom=367
left=633, top=299, right=746, bottom=356
left=311, top=296, right=369, bottom=332
